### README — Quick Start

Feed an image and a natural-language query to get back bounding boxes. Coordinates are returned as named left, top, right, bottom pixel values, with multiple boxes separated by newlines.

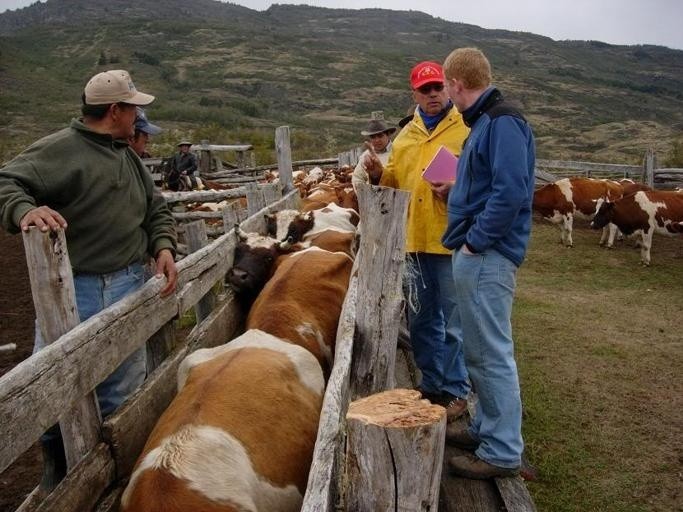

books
left=421, top=145, right=459, bottom=183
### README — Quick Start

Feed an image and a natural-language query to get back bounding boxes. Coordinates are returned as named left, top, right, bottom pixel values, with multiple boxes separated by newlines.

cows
left=245, top=245, right=360, bottom=372
left=223, top=221, right=295, bottom=295
left=117, top=326, right=326, bottom=512
left=623, top=183, right=654, bottom=195
left=264, top=209, right=316, bottom=248
left=590, top=189, right=682, bottom=268
left=186, top=160, right=362, bottom=227
left=618, top=177, right=634, bottom=186
left=301, top=201, right=360, bottom=259
left=532, top=175, right=625, bottom=251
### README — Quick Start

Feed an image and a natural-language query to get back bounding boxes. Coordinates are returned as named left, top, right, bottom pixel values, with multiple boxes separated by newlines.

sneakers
left=448, top=455, right=520, bottom=480
left=445, top=428, right=479, bottom=452
left=414, top=386, right=468, bottom=425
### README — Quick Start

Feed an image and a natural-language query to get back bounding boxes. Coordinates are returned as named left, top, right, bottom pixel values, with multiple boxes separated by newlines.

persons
left=351, top=121, right=394, bottom=212
left=360, top=60, right=472, bottom=424
left=122, top=102, right=161, bottom=157
left=170, top=136, right=199, bottom=189
left=438, top=42, right=539, bottom=481
left=0, top=70, right=180, bottom=496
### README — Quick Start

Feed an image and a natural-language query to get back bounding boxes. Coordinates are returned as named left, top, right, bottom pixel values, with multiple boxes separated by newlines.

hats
left=135, top=106, right=162, bottom=136
left=409, top=61, right=444, bottom=90
left=177, top=138, right=193, bottom=146
left=85, top=70, right=156, bottom=105
left=399, top=104, right=418, bottom=128
left=360, top=120, right=396, bottom=136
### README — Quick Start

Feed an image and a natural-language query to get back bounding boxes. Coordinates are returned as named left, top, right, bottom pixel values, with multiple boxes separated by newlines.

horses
left=160, top=160, right=203, bottom=191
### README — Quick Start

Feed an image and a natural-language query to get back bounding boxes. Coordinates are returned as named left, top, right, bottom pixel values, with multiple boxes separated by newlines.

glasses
left=418, top=83, right=443, bottom=93
